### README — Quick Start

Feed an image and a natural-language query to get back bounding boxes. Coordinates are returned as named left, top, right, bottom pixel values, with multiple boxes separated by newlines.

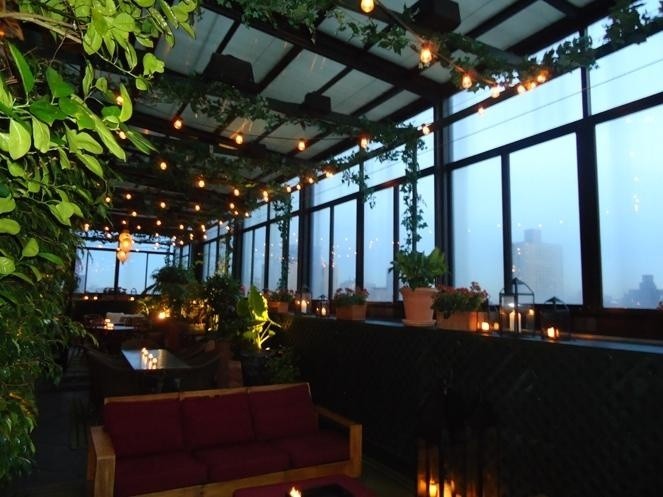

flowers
left=331, top=286, right=369, bottom=308
left=429, top=281, right=490, bottom=317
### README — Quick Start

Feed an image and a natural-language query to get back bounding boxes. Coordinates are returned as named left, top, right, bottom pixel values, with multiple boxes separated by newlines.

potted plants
left=392, top=245, right=448, bottom=328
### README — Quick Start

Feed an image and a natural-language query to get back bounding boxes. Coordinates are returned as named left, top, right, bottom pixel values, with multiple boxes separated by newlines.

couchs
left=86, top=379, right=366, bottom=497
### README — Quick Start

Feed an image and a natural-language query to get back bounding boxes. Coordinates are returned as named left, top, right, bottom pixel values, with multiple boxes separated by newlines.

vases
left=433, top=311, right=488, bottom=333
left=335, top=305, right=367, bottom=320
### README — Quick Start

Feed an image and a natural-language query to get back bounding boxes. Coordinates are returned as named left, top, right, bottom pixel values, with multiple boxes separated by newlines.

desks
left=62, top=311, right=242, bottom=448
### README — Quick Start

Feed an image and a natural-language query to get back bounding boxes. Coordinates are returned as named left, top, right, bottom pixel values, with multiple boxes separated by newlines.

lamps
left=537, top=297, right=572, bottom=340
left=292, top=282, right=330, bottom=316
left=353, top=0, right=554, bottom=95
left=413, top=419, right=502, bottom=496
left=496, top=278, right=537, bottom=339
